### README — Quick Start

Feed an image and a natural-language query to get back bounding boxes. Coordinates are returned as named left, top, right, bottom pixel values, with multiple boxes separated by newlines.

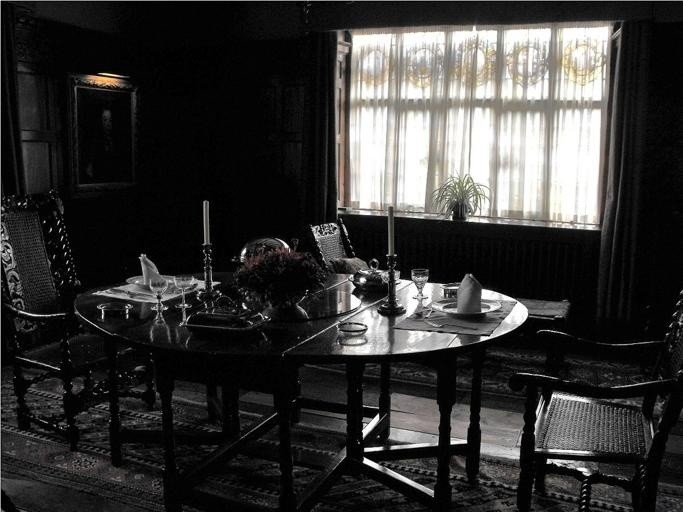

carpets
left=304, top=336, right=683, bottom=434
left=1, top=361, right=683, bottom=511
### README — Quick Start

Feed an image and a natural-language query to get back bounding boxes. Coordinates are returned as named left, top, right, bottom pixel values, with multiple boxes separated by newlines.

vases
left=263, top=291, right=309, bottom=320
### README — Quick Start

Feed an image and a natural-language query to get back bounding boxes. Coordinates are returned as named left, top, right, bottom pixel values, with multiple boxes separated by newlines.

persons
left=82, top=96, right=123, bottom=184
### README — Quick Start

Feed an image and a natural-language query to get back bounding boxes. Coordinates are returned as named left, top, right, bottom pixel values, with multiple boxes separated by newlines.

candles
left=203, top=201, right=210, bottom=244
left=387, top=205, right=395, bottom=255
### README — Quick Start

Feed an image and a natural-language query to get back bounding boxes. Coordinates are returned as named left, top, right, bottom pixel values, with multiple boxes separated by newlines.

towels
left=138, top=254, right=163, bottom=285
left=456, top=273, right=482, bottom=314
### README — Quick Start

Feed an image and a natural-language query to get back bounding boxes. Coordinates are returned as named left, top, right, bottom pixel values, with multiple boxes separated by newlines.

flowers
left=234, top=247, right=325, bottom=305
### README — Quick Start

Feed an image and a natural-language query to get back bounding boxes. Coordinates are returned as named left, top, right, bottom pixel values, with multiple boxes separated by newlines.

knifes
left=110, top=288, right=153, bottom=297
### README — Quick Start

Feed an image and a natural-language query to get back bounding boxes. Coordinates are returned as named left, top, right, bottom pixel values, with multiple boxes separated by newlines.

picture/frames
left=71, top=83, right=138, bottom=191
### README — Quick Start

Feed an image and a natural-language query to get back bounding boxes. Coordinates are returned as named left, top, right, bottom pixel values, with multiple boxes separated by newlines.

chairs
left=306, top=217, right=356, bottom=268
left=1, top=191, right=155, bottom=451
left=507, top=289, right=683, bottom=512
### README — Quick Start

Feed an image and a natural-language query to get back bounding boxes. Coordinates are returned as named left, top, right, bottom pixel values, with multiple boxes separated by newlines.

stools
left=516, top=298, right=571, bottom=372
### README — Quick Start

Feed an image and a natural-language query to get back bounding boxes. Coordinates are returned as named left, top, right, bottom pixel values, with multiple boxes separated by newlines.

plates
left=178, top=316, right=272, bottom=337
left=126, top=275, right=176, bottom=289
left=348, top=276, right=401, bottom=292
left=430, top=298, right=501, bottom=317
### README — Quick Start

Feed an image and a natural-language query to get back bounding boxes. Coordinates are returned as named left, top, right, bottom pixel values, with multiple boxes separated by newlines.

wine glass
left=148, top=278, right=168, bottom=311
left=175, top=309, right=192, bottom=339
left=174, top=275, right=194, bottom=309
left=150, top=312, right=170, bottom=343
left=411, top=269, right=429, bottom=300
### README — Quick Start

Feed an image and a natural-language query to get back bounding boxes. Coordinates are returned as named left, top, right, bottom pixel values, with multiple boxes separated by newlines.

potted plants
left=431, top=176, right=480, bottom=221
